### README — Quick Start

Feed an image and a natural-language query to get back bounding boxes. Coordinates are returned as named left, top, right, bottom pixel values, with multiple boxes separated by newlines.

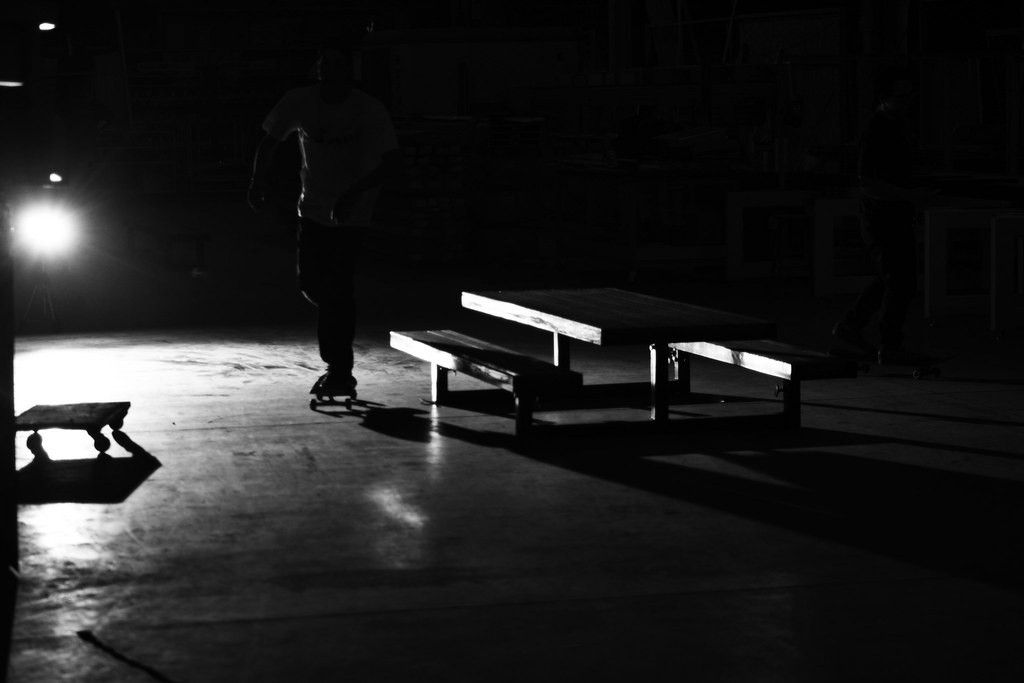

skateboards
left=309, top=374, right=358, bottom=409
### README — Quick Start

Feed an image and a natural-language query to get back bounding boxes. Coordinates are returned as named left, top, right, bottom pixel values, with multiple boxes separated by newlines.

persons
left=831, top=78, right=928, bottom=363
left=247, top=36, right=404, bottom=390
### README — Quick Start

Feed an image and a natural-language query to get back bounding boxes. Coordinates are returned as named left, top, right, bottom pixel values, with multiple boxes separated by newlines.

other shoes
left=319, top=371, right=351, bottom=388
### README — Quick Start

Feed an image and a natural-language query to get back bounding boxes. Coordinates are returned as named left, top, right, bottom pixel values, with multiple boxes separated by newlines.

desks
left=460, top=288, right=777, bottom=430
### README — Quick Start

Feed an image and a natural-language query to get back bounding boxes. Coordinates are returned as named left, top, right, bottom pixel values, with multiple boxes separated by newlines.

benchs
left=389, top=330, right=571, bottom=445
left=666, top=338, right=861, bottom=438
